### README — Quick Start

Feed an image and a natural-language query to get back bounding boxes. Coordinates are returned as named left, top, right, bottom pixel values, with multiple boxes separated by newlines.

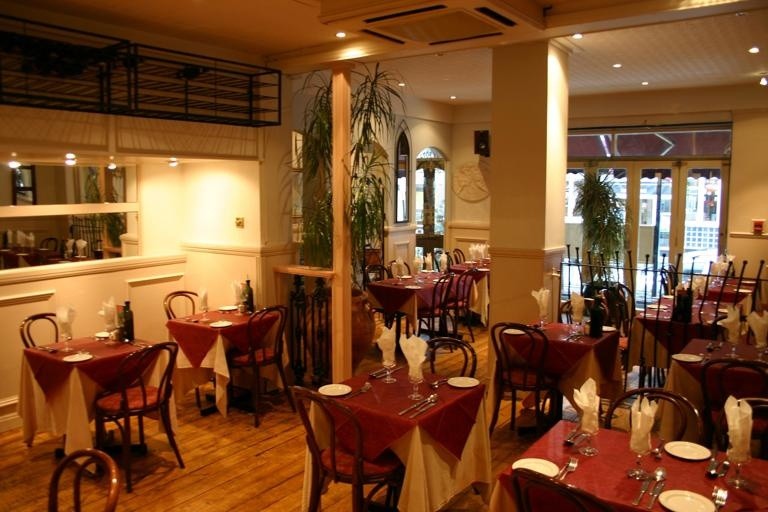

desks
left=167, top=309, right=281, bottom=417
left=18, top=336, right=163, bottom=477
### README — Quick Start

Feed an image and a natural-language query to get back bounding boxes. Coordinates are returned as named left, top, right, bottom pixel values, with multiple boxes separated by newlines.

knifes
left=397, top=393, right=439, bottom=419
left=630, top=479, right=665, bottom=509
left=563, top=419, right=587, bottom=444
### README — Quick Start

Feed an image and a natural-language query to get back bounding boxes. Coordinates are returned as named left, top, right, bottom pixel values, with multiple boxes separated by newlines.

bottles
left=245, top=279, right=254, bottom=312
left=590, top=295, right=605, bottom=338
left=585, top=320, right=591, bottom=335
left=124, top=300, right=135, bottom=340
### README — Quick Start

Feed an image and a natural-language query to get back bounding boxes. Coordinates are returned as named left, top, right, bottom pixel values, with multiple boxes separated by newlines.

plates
left=707, top=320, right=724, bottom=326
left=671, top=353, right=704, bottom=362
left=718, top=308, right=731, bottom=313
left=209, top=319, right=233, bottom=328
left=503, top=325, right=525, bottom=335
left=658, top=489, right=716, bottom=512
left=217, top=305, right=237, bottom=311
left=742, top=280, right=756, bottom=284
left=318, top=384, right=352, bottom=396
left=398, top=268, right=449, bottom=290
left=601, top=326, right=617, bottom=331
left=733, top=288, right=752, bottom=293
left=661, top=294, right=675, bottom=299
left=664, top=440, right=714, bottom=461
left=511, top=457, right=559, bottom=479
left=94, top=331, right=110, bottom=338
left=447, top=376, right=480, bottom=388
left=646, top=304, right=668, bottom=309
left=464, top=256, right=489, bottom=272
left=62, top=352, right=94, bottom=363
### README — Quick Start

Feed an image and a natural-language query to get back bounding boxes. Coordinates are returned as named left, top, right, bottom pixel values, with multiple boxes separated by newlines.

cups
left=752, top=219, right=766, bottom=234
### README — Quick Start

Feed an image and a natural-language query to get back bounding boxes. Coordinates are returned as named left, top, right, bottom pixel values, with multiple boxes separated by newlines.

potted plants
left=570, top=164, right=628, bottom=324
left=285, top=61, right=416, bottom=389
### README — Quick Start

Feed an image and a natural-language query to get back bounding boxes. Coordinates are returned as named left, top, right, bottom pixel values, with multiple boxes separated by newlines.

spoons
left=651, top=440, right=667, bottom=459
left=342, top=384, right=372, bottom=399
left=706, top=455, right=730, bottom=479
left=647, top=464, right=667, bottom=496
left=429, top=376, right=448, bottom=389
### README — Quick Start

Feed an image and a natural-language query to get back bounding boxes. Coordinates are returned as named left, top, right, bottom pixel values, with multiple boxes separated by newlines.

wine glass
left=537, top=309, right=548, bottom=330
left=407, top=365, right=425, bottom=401
left=470, top=246, right=485, bottom=268
left=578, top=416, right=600, bottom=457
left=626, top=429, right=653, bottom=480
left=724, top=327, right=743, bottom=361
left=198, top=300, right=211, bottom=322
left=571, top=316, right=584, bottom=342
left=726, top=439, right=752, bottom=488
left=754, top=337, right=767, bottom=362
left=382, top=351, right=396, bottom=384
left=394, top=256, right=446, bottom=285
left=59, top=324, right=74, bottom=352
left=103, top=319, right=118, bottom=345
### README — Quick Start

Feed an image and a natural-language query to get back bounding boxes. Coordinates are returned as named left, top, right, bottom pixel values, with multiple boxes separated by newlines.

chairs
left=163, top=291, right=218, bottom=409
left=95, top=342, right=185, bottom=491
left=288, top=246, right=767, bottom=511
left=38, top=237, right=103, bottom=259
left=46, top=449, right=121, bottom=510
left=223, top=304, right=296, bottom=425
left=19, top=312, right=62, bottom=348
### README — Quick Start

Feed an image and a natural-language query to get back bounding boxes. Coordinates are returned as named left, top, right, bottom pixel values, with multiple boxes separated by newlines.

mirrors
left=394, top=130, right=410, bottom=225
left=0, top=161, right=138, bottom=269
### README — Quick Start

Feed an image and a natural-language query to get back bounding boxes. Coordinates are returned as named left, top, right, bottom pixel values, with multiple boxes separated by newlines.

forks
left=712, top=487, right=729, bottom=511
left=559, top=457, right=579, bottom=482
